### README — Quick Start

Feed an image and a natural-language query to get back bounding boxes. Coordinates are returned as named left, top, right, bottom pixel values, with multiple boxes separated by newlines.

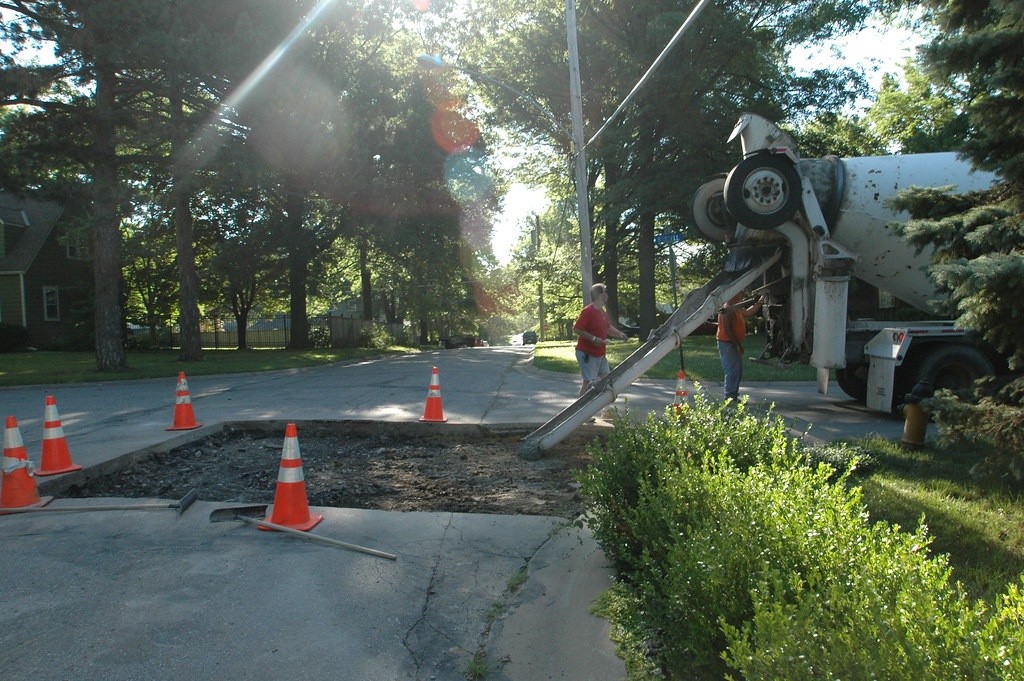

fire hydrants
left=895, top=370, right=938, bottom=453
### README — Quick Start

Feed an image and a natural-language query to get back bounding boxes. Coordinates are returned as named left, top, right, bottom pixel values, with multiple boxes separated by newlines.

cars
left=522, top=332, right=539, bottom=346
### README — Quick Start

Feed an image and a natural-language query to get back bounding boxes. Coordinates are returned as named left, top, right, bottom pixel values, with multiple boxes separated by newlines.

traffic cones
left=0, top=414, right=55, bottom=515
left=257, top=422, right=323, bottom=533
left=32, top=394, right=83, bottom=477
left=671, top=369, right=690, bottom=416
left=419, top=365, right=448, bottom=422
left=164, top=371, right=204, bottom=430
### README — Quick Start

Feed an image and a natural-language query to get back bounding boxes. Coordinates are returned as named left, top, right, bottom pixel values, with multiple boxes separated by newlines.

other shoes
left=600, top=410, right=624, bottom=418
left=588, top=417, right=595, bottom=422
left=736, top=398, right=747, bottom=405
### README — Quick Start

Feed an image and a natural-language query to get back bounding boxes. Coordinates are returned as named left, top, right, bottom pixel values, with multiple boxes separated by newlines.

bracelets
left=592, top=336, right=596, bottom=344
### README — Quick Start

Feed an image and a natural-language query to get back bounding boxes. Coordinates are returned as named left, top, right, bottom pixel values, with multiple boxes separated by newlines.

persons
left=716, top=290, right=764, bottom=407
left=573, top=284, right=628, bottom=422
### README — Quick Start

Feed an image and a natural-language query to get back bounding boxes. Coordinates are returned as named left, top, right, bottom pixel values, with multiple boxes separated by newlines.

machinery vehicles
left=516, top=110, right=1024, bottom=459
